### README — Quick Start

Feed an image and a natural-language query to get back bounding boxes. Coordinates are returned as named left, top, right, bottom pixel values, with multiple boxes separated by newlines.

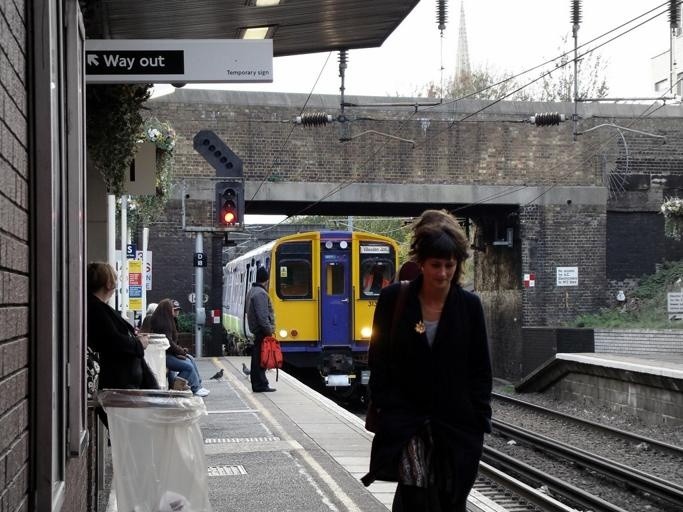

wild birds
left=209, top=369, right=224, bottom=382
left=242, top=363, right=250, bottom=379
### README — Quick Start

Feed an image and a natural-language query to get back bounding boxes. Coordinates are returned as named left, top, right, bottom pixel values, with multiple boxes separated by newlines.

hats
left=170, top=300, right=183, bottom=310
left=256, top=266, right=270, bottom=283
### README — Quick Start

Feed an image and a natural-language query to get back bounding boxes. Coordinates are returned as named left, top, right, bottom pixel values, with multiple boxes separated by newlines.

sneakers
left=194, top=388, right=210, bottom=396
left=253, top=385, right=277, bottom=393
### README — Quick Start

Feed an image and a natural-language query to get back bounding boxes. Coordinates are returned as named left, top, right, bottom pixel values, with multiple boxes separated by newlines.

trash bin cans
left=136, top=332, right=171, bottom=389
left=100, top=387, right=214, bottom=512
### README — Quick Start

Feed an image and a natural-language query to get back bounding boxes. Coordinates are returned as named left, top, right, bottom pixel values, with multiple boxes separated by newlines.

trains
left=221, top=227, right=402, bottom=413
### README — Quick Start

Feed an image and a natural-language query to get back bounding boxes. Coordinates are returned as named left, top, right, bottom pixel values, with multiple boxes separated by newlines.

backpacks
left=260, top=334, right=283, bottom=369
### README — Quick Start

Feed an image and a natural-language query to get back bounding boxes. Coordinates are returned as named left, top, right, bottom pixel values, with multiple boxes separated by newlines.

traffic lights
left=212, top=180, right=245, bottom=226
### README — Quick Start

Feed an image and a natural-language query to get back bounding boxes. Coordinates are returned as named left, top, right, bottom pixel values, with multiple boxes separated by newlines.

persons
left=167, top=299, right=191, bottom=388
left=139, top=298, right=209, bottom=397
left=135, top=302, right=157, bottom=327
left=361, top=260, right=389, bottom=291
left=86, top=262, right=161, bottom=431
left=245, top=266, right=283, bottom=392
left=359, top=211, right=495, bottom=511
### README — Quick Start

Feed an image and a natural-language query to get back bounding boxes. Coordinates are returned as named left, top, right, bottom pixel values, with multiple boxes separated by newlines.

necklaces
left=419, top=297, right=441, bottom=313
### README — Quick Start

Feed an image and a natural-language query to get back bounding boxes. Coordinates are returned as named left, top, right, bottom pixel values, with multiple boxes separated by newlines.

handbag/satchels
left=361, top=419, right=436, bottom=489
